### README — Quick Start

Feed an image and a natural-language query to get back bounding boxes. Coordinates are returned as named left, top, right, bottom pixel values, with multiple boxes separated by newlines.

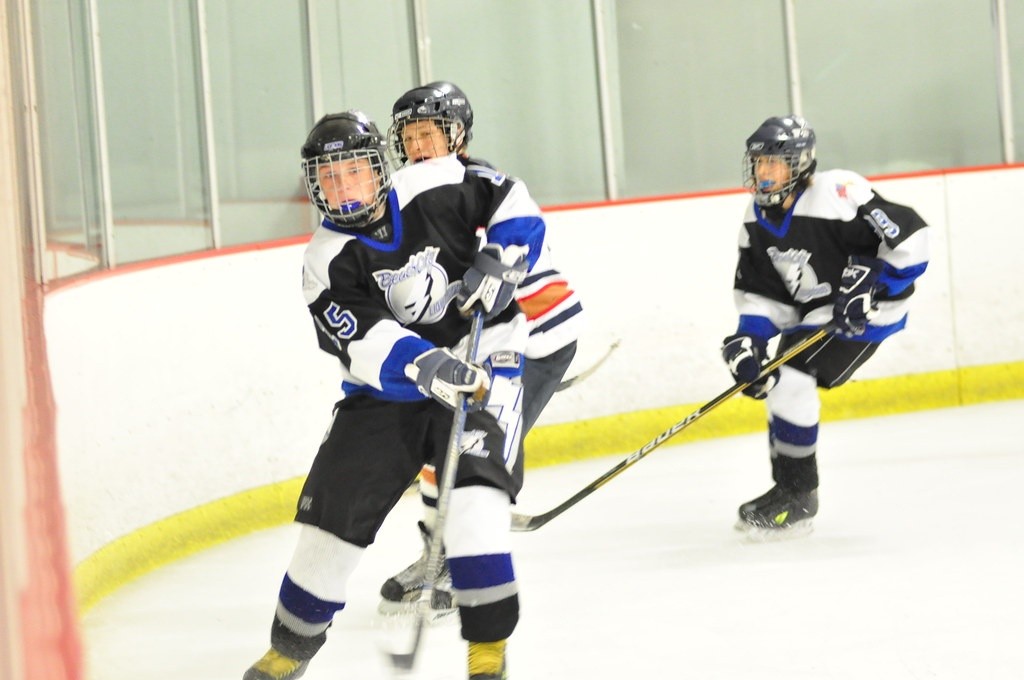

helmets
left=387, top=81, right=475, bottom=170
left=300, top=110, right=392, bottom=234
left=741, top=114, right=817, bottom=218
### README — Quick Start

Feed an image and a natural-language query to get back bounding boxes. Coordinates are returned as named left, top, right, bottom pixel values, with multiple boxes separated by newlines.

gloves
left=720, top=332, right=780, bottom=399
left=457, top=243, right=530, bottom=321
left=404, top=347, right=492, bottom=412
left=832, top=254, right=883, bottom=341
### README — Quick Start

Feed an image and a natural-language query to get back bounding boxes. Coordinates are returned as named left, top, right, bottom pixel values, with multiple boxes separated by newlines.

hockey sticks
left=385, top=298, right=841, bottom=674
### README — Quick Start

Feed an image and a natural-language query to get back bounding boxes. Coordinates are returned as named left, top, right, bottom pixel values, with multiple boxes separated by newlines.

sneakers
left=732, top=484, right=819, bottom=541
left=373, top=521, right=457, bottom=624
left=242, top=645, right=310, bottom=680
left=465, top=640, right=508, bottom=680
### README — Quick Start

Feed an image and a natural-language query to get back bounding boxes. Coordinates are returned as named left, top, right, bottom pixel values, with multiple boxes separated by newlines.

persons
left=239, top=111, right=543, bottom=680
left=717, top=113, right=929, bottom=544
left=377, top=80, right=585, bottom=629
